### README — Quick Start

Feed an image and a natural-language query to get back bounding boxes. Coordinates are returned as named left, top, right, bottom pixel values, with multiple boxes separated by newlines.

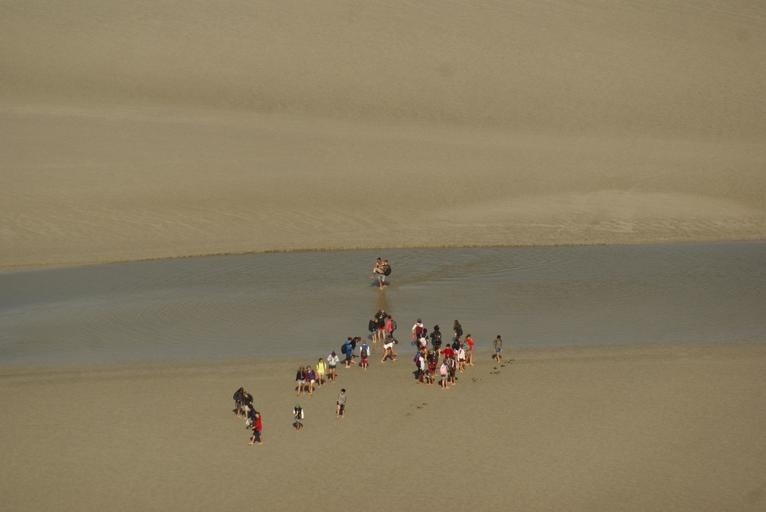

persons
left=360, top=340, right=369, bottom=369
left=369, top=310, right=398, bottom=363
left=495, top=334, right=503, bottom=363
left=368, top=257, right=391, bottom=290
left=296, top=336, right=358, bottom=394
left=412, top=319, right=475, bottom=389
left=233, top=386, right=263, bottom=445
left=293, top=403, right=304, bottom=432
left=336, top=388, right=346, bottom=418
